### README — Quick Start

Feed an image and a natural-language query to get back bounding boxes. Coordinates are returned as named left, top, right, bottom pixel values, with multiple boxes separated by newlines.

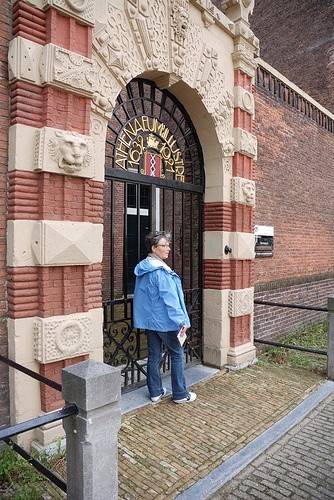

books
left=176, top=328, right=188, bottom=347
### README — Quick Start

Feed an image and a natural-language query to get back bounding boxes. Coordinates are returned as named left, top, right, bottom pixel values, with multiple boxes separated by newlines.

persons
left=132, top=230, right=198, bottom=404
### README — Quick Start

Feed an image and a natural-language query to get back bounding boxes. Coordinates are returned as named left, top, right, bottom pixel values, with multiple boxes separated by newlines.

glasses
left=155, top=243, right=170, bottom=248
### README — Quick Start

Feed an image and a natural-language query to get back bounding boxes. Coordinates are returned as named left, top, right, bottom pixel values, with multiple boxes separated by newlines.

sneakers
left=173, top=392, right=197, bottom=404
left=150, top=387, right=166, bottom=401
